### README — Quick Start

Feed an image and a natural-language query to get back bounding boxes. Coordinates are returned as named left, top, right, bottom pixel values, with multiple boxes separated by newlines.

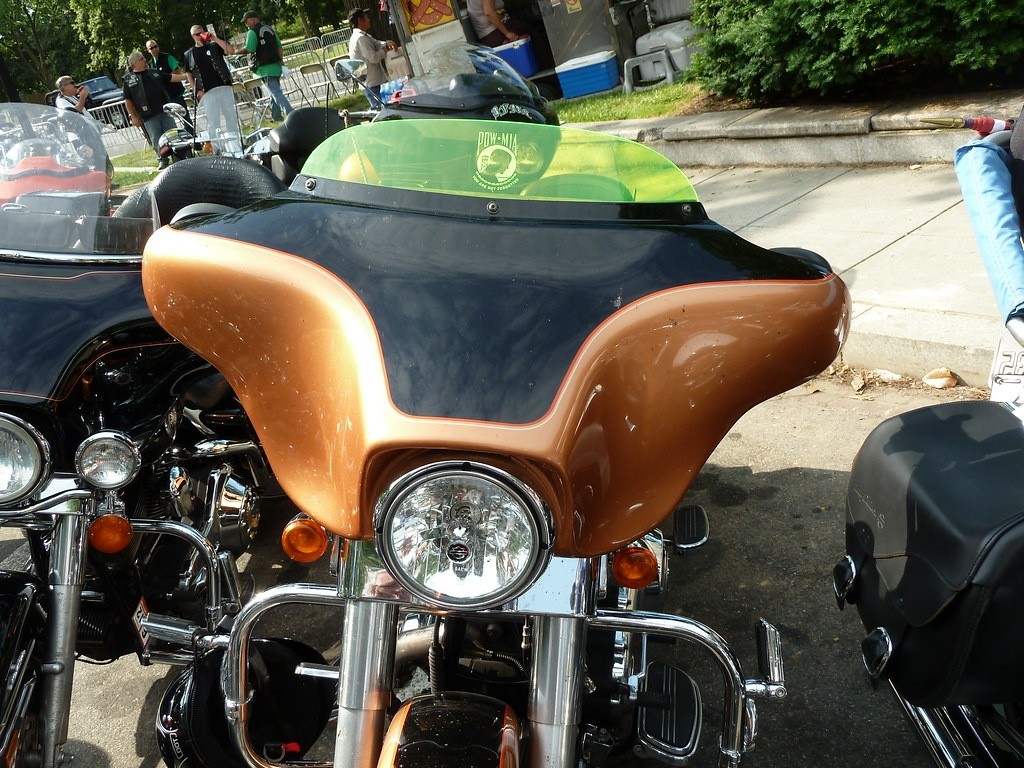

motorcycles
left=831, top=301, right=1024, bottom=768
left=136, top=120, right=850, bottom=768
left=0, top=38, right=640, bottom=768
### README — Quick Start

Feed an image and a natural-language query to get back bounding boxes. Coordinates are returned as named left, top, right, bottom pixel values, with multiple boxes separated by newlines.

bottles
left=380, top=76, right=405, bottom=102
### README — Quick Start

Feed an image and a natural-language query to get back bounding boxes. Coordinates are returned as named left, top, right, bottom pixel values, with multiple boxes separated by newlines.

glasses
left=148, top=45, right=158, bottom=51
left=192, top=31, right=203, bottom=37
left=62, top=81, right=74, bottom=86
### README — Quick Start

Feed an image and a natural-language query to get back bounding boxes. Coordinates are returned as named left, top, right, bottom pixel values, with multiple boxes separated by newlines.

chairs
left=243, top=76, right=285, bottom=124
left=183, top=98, right=210, bottom=132
left=330, top=55, right=360, bottom=96
left=300, top=64, right=340, bottom=107
left=232, top=82, right=268, bottom=128
left=279, top=74, right=311, bottom=116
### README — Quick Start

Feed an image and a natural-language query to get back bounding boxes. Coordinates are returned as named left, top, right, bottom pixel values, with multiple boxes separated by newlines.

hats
left=128, top=52, right=144, bottom=66
left=348, top=6, right=370, bottom=24
left=240, top=11, right=258, bottom=25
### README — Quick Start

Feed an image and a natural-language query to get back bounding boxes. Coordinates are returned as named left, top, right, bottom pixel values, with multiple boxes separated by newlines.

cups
left=200, top=32, right=210, bottom=41
left=79, top=86, right=88, bottom=98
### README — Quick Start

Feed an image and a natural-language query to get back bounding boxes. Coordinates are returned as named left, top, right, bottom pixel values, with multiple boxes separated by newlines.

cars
left=41, top=76, right=132, bottom=129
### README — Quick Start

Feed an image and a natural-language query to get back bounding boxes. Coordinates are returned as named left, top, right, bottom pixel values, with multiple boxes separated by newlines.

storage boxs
left=555, top=50, right=620, bottom=100
left=478, top=35, right=538, bottom=83
left=828, top=401, right=1024, bottom=711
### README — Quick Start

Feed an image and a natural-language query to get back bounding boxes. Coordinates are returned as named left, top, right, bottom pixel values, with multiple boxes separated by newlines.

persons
left=183, top=25, right=249, bottom=157
left=56, top=76, right=112, bottom=177
left=123, top=53, right=187, bottom=169
left=0, top=113, right=19, bottom=143
left=348, top=8, right=398, bottom=107
left=467, top=0, right=518, bottom=47
left=146, top=40, right=203, bottom=153
left=236, top=11, right=294, bottom=122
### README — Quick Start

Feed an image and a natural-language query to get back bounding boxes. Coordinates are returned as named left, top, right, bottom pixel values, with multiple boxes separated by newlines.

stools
left=622, top=48, right=682, bottom=95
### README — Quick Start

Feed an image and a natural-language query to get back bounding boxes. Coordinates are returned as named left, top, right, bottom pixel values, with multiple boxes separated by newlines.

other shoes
left=158, top=161, right=168, bottom=169
left=110, top=183, right=120, bottom=190
